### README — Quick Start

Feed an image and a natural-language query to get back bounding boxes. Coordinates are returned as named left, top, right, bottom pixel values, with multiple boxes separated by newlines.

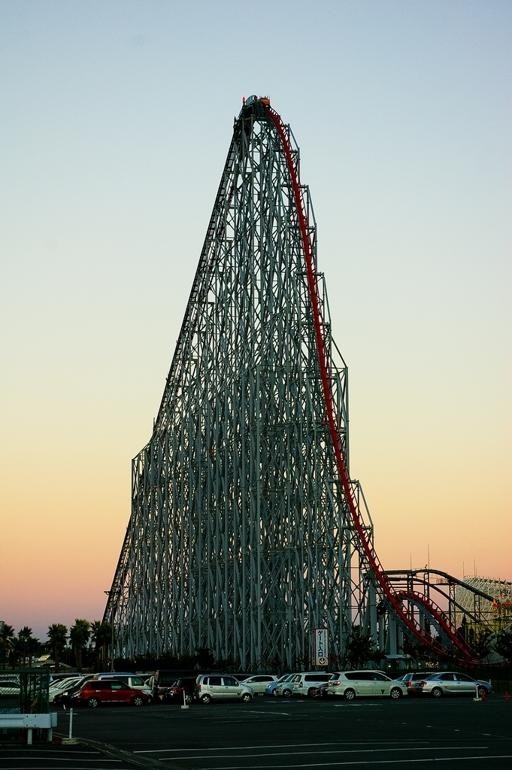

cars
left=265, top=673, right=296, bottom=700
left=240, top=675, right=280, bottom=695
left=197, top=674, right=254, bottom=706
left=416, top=672, right=493, bottom=698
left=79, top=679, right=151, bottom=707
left=401, top=672, right=438, bottom=693
left=1, top=670, right=199, bottom=706
left=292, top=673, right=334, bottom=700
left=327, top=670, right=408, bottom=701
